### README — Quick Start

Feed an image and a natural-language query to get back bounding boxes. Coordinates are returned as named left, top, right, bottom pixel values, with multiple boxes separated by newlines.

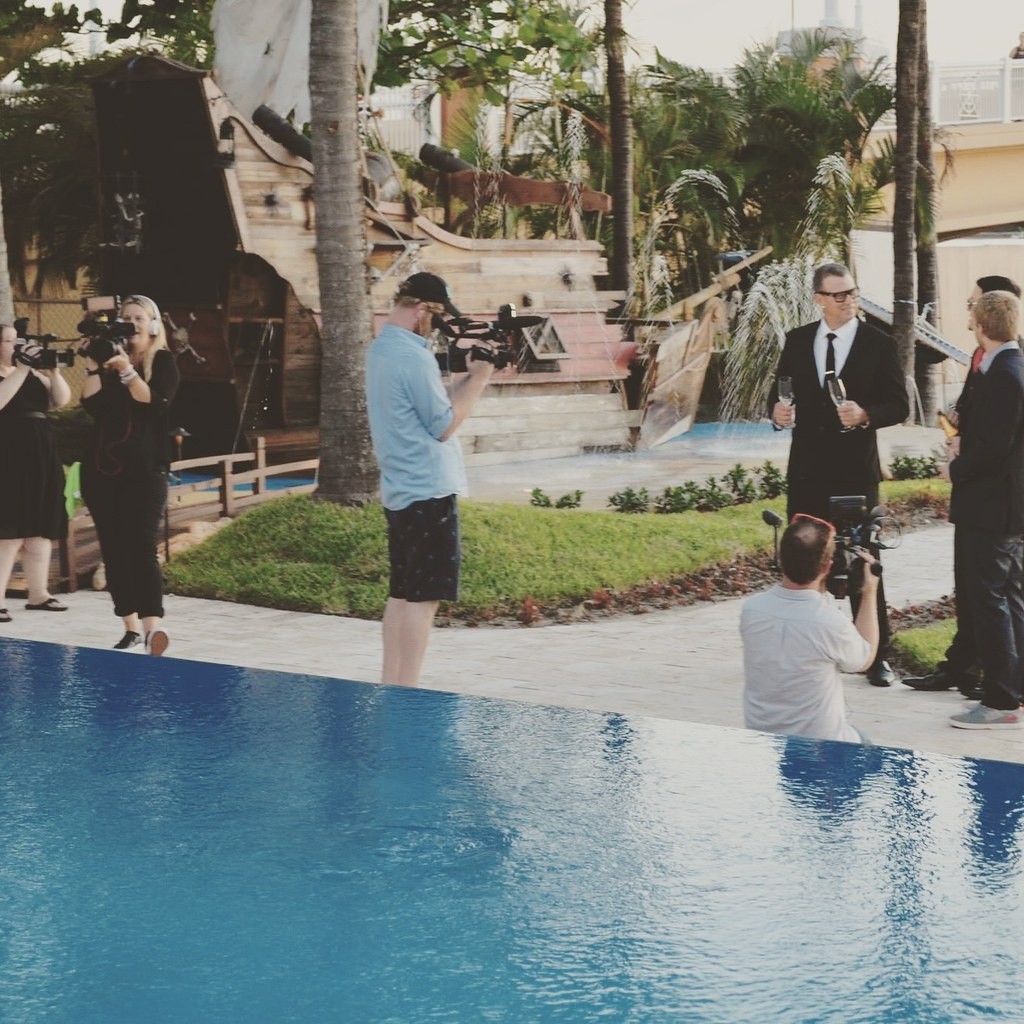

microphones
left=487, top=315, right=544, bottom=330
left=869, top=505, right=886, bottom=538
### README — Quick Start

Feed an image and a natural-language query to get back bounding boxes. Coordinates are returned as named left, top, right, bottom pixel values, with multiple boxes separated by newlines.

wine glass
left=779, top=377, right=796, bottom=429
left=827, top=378, right=856, bottom=433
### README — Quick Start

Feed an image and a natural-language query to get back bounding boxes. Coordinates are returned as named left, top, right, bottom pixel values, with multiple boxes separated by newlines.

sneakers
left=948, top=702, right=1022, bottom=729
left=144, top=629, right=169, bottom=656
left=113, top=631, right=145, bottom=651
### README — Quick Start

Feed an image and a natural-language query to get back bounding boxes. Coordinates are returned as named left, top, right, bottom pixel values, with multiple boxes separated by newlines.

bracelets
left=84, top=367, right=100, bottom=376
left=119, top=365, right=138, bottom=384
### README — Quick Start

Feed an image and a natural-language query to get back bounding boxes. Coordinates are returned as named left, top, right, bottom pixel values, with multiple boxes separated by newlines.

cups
left=931, top=443, right=949, bottom=462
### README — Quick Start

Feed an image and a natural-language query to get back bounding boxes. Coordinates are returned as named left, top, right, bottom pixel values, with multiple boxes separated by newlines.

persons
left=740, top=518, right=880, bottom=744
left=365, top=272, right=496, bottom=687
left=901, top=276, right=1021, bottom=699
left=767, top=263, right=910, bottom=686
left=77, top=295, right=186, bottom=656
left=938, top=289, right=1024, bottom=730
left=0, top=322, right=71, bottom=623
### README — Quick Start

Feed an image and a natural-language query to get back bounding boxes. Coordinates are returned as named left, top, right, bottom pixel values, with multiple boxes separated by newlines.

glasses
left=967, top=299, right=979, bottom=309
left=815, top=287, right=858, bottom=303
left=791, top=513, right=835, bottom=556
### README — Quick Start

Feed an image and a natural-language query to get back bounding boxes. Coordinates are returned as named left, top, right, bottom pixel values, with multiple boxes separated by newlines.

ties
left=971, top=347, right=983, bottom=372
left=824, top=333, right=837, bottom=392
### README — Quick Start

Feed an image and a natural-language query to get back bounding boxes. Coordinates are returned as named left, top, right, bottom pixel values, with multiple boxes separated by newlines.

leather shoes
left=868, top=660, right=892, bottom=685
left=961, top=682, right=987, bottom=697
left=901, top=671, right=956, bottom=690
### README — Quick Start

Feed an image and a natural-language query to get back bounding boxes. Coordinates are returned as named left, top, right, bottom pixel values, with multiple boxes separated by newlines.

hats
left=398, top=272, right=461, bottom=319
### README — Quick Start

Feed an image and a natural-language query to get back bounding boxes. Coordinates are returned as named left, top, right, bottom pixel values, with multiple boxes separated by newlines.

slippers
left=24, top=598, right=67, bottom=611
left=0, top=609, right=11, bottom=622
